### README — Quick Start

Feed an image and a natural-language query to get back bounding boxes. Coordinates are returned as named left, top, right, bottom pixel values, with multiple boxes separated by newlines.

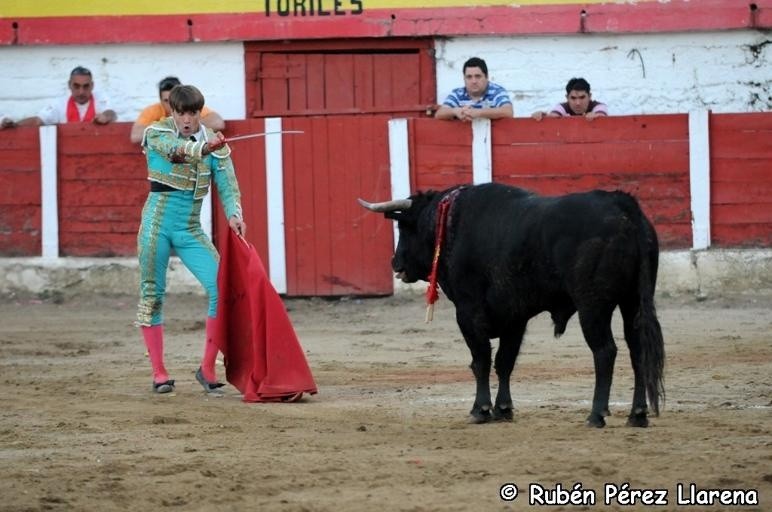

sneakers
left=152, top=375, right=175, bottom=392
left=195, top=367, right=225, bottom=395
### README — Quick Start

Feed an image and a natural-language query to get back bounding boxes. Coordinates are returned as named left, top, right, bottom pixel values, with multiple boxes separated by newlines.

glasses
left=159, top=77, right=180, bottom=90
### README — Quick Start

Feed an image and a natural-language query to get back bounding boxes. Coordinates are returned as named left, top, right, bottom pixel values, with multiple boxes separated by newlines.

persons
left=136, top=84, right=248, bottom=394
left=433, top=56, right=515, bottom=124
left=0, top=65, right=117, bottom=130
left=531, top=76, right=608, bottom=121
left=129, top=75, right=226, bottom=143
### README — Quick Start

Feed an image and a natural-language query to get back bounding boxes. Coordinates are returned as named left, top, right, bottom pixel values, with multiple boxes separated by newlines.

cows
left=357, top=182, right=665, bottom=428
